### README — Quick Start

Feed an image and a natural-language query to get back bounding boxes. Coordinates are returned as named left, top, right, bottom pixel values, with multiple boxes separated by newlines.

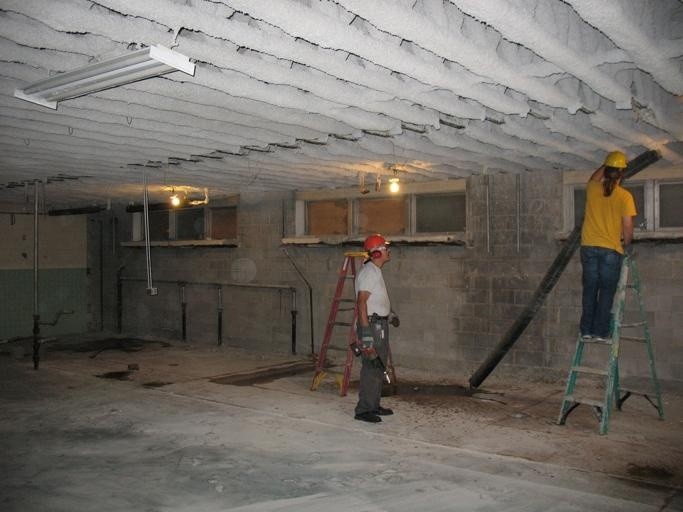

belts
left=368, top=316, right=387, bottom=320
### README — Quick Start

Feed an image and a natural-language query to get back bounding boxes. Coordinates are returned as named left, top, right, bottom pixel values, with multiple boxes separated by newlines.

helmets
left=363, top=235, right=390, bottom=252
left=605, top=151, right=627, bottom=168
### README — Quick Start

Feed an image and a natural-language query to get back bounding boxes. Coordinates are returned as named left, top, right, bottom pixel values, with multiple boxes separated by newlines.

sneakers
left=354, top=413, right=380, bottom=422
left=584, top=335, right=610, bottom=341
left=373, top=407, right=392, bottom=415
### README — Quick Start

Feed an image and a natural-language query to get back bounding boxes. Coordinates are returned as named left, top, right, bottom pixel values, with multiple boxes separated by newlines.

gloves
left=362, top=326, right=373, bottom=348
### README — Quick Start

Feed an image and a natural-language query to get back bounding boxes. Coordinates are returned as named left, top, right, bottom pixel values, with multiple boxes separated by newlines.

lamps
left=11, top=44, right=197, bottom=111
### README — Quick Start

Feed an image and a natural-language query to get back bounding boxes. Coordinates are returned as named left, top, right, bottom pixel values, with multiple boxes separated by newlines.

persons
left=578, top=151, right=638, bottom=341
left=353, top=233, right=400, bottom=423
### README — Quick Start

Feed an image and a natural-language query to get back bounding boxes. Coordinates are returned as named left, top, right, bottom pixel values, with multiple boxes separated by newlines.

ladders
left=556, top=246, right=664, bottom=435
left=310, top=252, right=397, bottom=397
